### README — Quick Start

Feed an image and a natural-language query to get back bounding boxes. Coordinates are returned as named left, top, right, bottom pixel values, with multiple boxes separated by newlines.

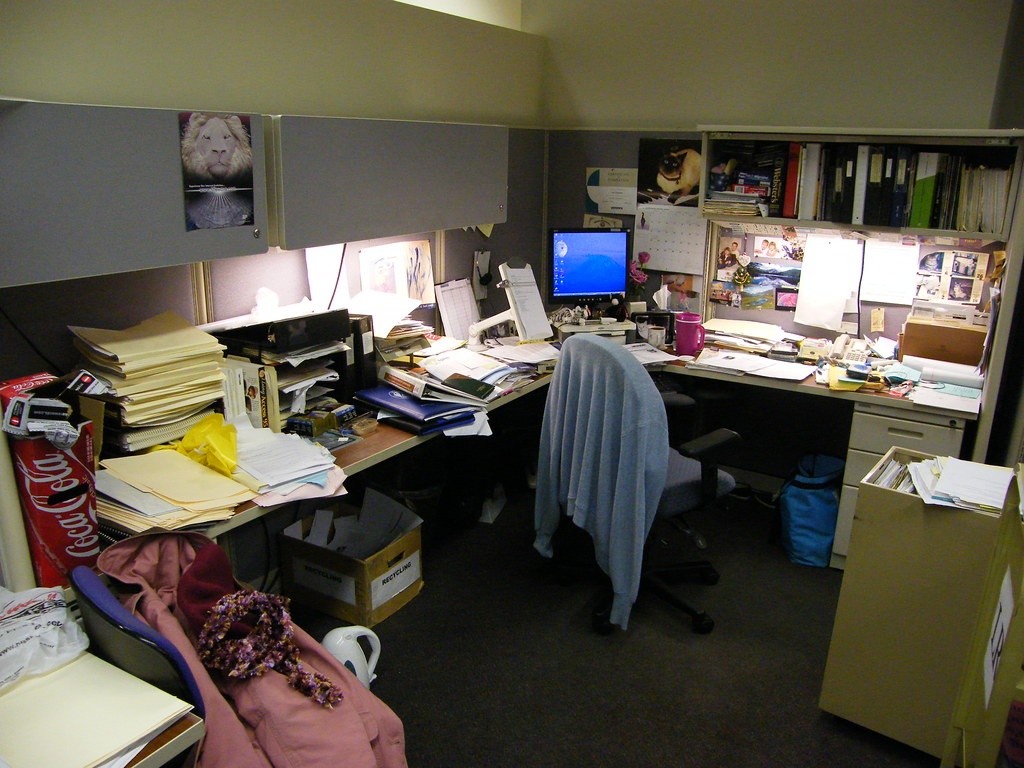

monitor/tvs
left=546, top=227, right=632, bottom=322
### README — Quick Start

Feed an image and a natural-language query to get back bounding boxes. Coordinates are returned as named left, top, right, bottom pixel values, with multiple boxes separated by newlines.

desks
left=200, top=369, right=554, bottom=548
left=0, top=652, right=206, bottom=768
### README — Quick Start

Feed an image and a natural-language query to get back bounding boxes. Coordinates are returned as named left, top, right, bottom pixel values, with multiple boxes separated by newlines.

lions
left=179, top=112, right=253, bottom=180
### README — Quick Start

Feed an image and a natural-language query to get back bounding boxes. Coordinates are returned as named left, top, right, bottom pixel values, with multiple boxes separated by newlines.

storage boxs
left=816, top=444, right=1010, bottom=758
left=283, top=487, right=425, bottom=629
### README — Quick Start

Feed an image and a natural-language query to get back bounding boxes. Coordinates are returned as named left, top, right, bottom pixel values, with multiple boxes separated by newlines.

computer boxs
left=549, top=317, right=636, bottom=346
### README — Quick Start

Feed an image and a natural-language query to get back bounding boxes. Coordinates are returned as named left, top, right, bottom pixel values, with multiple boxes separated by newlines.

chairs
left=533, top=334, right=740, bottom=636
left=70, top=566, right=205, bottom=723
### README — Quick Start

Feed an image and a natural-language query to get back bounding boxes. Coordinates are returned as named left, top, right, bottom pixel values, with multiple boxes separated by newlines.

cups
left=676, top=313, right=705, bottom=355
left=648, top=326, right=666, bottom=349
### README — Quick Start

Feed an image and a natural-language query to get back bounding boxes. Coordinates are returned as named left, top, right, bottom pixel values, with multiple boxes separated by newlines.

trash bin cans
left=275, top=501, right=424, bottom=627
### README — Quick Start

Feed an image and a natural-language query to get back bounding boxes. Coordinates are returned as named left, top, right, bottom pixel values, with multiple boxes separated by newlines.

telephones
left=830, top=334, right=872, bottom=368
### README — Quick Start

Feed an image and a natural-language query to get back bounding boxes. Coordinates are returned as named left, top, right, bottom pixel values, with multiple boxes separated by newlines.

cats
left=655, top=148, right=701, bottom=204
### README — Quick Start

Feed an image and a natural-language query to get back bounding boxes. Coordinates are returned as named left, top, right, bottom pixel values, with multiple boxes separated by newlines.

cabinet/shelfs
left=645, top=345, right=983, bottom=571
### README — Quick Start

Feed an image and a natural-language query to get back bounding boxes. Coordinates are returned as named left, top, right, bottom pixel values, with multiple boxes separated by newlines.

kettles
left=319, top=625, right=381, bottom=692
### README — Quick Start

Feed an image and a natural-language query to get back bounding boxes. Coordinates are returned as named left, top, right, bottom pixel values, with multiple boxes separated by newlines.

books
left=700, top=175, right=775, bottom=216
left=349, top=287, right=487, bottom=431
left=68, top=308, right=227, bottom=451
left=871, top=446, right=1012, bottom=521
left=768, top=143, right=1010, bottom=232
